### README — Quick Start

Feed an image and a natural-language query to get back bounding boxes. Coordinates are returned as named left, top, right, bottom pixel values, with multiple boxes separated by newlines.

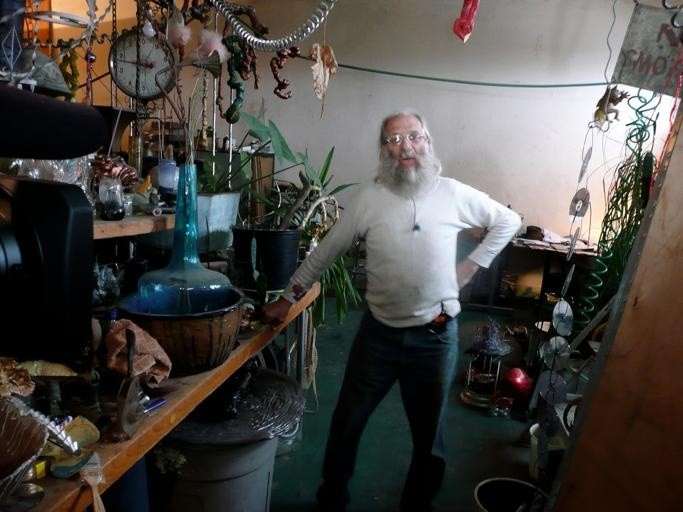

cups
left=98, top=178, right=124, bottom=222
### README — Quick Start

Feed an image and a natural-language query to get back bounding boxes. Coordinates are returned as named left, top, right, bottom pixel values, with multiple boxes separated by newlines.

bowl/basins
left=0, top=397, right=49, bottom=504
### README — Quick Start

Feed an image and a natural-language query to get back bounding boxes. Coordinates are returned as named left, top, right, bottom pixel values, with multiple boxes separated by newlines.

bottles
left=159, top=161, right=176, bottom=201
left=122, top=194, right=134, bottom=217
left=194, top=124, right=235, bottom=152
left=250, top=141, right=259, bottom=144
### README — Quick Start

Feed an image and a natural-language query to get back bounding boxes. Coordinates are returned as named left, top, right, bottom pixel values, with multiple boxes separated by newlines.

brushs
left=81, top=453, right=105, bottom=512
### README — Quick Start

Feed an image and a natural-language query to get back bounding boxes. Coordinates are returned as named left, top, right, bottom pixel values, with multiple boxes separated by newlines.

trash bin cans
left=473, top=477, right=549, bottom=512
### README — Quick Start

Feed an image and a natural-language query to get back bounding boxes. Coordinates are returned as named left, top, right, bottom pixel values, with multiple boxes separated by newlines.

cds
left=538, top=371, right=567, bottom=406
left=569, top=188, right=590, bottom=224
left=567, top=227, right=580, bottom=260
left=561, top=265, right=576, bottom=296
left=578, top=147, right=592, bottom=183
left=544, top=336, right=570, bottom=370
left=553, top=301, right=574, bottom=336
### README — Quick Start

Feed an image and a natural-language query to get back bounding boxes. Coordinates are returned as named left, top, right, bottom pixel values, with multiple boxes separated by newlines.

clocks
left=105, top=22, right=182, bottom=102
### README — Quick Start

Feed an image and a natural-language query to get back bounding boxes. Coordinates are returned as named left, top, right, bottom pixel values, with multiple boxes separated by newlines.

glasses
left=384, top=133, right=427, bottom=147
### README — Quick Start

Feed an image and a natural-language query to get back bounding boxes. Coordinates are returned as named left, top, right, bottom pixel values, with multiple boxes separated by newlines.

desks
left=2, top=269, right=322, bottom=512
left=464, top=233, right=602, bottom=318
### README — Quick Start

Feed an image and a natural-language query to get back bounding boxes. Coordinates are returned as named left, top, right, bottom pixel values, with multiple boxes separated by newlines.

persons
left=257, top=110, right=523, bottom=512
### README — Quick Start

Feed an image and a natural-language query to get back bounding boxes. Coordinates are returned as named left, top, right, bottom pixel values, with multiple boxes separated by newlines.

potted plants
left=191, top=111, right=317, bottom=255
left=227, top=146, right=366, bottom=329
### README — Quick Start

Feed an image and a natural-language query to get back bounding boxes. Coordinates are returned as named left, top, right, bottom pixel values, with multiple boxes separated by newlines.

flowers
left=144, top=15, right=234, bottom=162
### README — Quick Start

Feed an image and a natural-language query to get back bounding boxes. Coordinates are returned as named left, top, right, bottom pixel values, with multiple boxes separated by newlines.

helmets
left=22, top=48, right=73, bottom=97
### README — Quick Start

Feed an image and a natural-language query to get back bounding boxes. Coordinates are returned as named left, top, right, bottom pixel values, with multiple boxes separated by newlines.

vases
left=134, top=143, right=242, bottom=314
